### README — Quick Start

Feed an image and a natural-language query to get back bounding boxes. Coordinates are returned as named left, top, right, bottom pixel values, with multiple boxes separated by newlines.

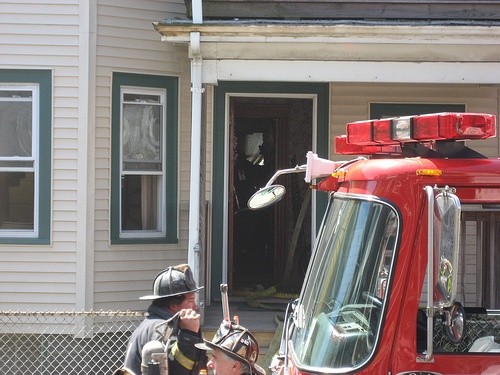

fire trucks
left=247, top=112, right=499, bottom=375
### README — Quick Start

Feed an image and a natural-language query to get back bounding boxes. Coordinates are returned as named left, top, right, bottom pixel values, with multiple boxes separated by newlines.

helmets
left=194, top=320, right=266, bottom=375
left=139, top=264, right=204, bottom=300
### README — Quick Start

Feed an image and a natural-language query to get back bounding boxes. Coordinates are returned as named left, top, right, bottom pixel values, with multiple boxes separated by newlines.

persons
left=112, top=263, right=209, bottom=375
left=194, top=320, right=267, bottom=375
